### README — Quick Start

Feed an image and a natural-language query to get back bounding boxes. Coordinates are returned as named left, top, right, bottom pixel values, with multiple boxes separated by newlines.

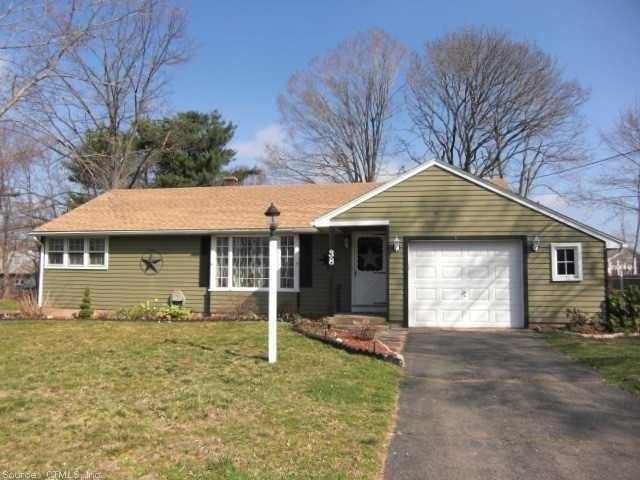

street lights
left=261, top=202, right=282, bottom=362
